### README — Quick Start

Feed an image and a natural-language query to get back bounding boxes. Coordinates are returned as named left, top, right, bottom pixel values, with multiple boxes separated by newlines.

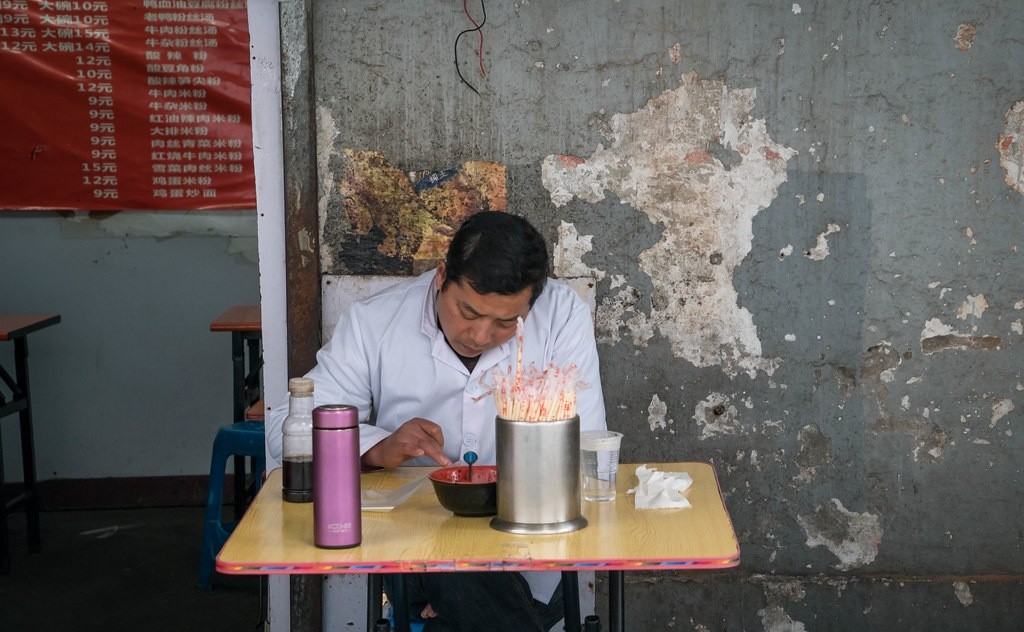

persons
left=266, top=208, right=609, bottom=632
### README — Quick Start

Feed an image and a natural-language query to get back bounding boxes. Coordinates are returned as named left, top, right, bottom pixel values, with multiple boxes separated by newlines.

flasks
left=312, top=404, right=362, bottom=549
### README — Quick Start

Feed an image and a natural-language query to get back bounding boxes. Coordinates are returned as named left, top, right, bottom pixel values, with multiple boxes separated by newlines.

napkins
left=626, top=463, right=693, bottom=511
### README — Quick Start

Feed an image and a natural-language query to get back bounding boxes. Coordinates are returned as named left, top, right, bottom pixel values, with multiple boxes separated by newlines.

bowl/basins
left=427, top=466, right=497, bottom=516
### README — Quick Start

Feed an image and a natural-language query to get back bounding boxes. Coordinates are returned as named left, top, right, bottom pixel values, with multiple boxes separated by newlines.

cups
left=578, top=431, right=624, bottom=502
left=489, top=413, right=588, bottom=534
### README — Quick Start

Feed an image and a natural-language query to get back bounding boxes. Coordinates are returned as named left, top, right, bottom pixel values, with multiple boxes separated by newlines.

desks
left=210, top=304, right=263, bottom=520
left=0, top=314, right=60, bottom=577
left=216, top=461, right=740, bottom=632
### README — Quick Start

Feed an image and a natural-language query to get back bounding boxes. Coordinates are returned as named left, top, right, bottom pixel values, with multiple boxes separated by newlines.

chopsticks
left=472, top=316, right=592, bottom=422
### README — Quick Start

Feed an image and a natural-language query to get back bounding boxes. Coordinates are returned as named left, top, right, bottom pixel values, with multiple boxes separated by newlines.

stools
left=200, top=423, right=265, bottom=590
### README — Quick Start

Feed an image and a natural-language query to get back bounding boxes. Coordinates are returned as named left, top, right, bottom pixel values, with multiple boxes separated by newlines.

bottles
left=282, top=378, right=315, bottom=503
